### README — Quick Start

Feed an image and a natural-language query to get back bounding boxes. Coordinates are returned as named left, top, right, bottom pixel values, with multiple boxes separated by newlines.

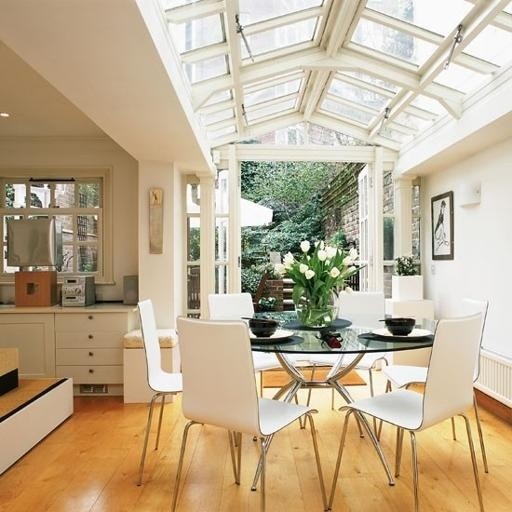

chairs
left=329, top=312, right=485, bottom=511
left=137, top=290, right=490, bottom=491
left=170, top=316, right=330, bottom=511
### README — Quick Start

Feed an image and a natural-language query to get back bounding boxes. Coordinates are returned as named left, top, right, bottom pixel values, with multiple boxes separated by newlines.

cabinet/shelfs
left=55, top=312, right=141, bottom=395
left=1, top=313, right=58, bottom=377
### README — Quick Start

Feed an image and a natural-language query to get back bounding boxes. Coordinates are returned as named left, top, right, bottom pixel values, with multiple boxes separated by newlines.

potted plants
left=391, top=255, right=422, bottom=301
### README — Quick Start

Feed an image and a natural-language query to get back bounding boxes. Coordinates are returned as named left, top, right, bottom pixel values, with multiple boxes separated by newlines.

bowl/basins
left=249, top=319, right=278, bottom=338
left=385, top=318, right=415, bottom=336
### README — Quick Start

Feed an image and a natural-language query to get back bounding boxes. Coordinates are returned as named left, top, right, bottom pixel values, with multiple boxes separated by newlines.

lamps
left=444, top=175, right=482, bottom=206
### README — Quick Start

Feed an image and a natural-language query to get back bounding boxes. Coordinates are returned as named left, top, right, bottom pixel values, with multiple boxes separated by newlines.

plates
left=372, top=328, right=432, bottom=339
left=248, top=328, right=294, bottom=341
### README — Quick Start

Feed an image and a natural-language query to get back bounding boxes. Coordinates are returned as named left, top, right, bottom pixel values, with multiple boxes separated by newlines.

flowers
left=272, top=237, right=366, bottom=323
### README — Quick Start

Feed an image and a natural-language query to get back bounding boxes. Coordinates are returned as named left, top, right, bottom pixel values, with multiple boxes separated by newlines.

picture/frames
left=430, top=190, right=454, bottom=260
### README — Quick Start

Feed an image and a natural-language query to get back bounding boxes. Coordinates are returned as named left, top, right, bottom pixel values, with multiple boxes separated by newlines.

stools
left=124, top=329, right=180, bottom=404
